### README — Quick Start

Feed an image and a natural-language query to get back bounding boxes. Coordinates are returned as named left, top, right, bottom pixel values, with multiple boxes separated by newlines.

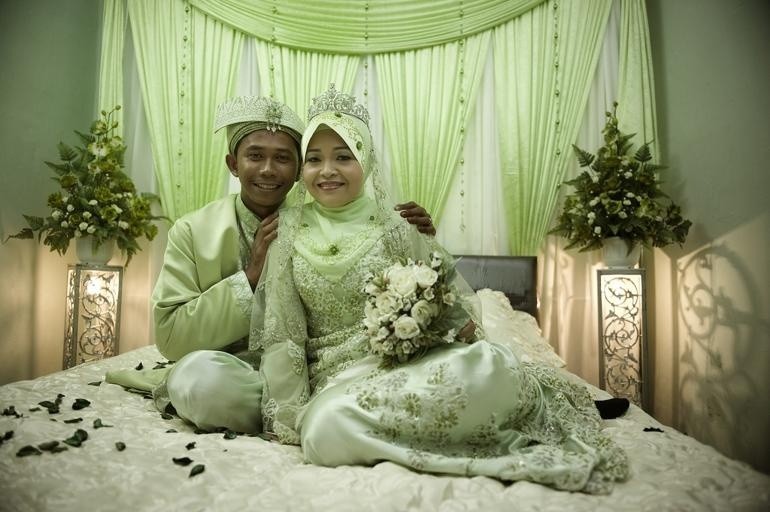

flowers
left=547, top=100, right=693, bottom=254
left=360, top=250, right=471, bottom=369
left=1, top=104, right=174, bottom=271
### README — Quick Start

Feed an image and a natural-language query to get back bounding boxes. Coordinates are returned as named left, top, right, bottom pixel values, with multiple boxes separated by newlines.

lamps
left=63, top=264, right=123, bottom=371
left=597, top=270, right=647, bottom=414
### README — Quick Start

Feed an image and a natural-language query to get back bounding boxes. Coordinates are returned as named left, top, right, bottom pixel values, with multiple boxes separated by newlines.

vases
left=76, top=236, right=115, bottom=266
left=602, top=236, right=641, bottom=270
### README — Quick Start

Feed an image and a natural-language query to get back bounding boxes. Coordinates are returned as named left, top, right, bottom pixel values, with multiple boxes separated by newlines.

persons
left=150, top=95, right=436, bottom=435
left=249, top=85, right=632, bottom=496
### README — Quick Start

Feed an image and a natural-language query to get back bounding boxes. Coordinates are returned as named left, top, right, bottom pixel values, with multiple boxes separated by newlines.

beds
left=0, top=256, right=770, bottom=512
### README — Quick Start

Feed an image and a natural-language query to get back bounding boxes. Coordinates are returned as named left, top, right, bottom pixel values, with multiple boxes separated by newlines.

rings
left=428, top=217, right=432, bottom=225
